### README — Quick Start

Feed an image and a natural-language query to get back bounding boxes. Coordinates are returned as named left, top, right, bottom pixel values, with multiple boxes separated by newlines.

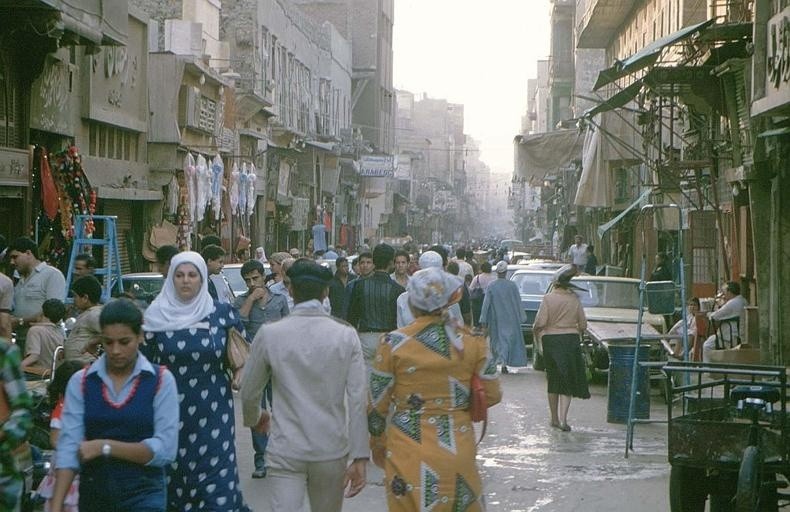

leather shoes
left=558, top=422, right=571, bottom=432
left=252, top=471, right=265, bottom=478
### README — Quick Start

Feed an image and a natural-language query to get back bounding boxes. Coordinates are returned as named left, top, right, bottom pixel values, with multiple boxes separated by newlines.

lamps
left=199, top=72, right=205, bottom=85
left=218, top=83, right=224, bottom=95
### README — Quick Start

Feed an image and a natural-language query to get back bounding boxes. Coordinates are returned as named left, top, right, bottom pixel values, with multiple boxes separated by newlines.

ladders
left=63, top=216, right=124, bottom=305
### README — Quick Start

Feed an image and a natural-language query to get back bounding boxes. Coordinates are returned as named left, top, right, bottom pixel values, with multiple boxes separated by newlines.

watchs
left=102, top=438, right=112, bottom=456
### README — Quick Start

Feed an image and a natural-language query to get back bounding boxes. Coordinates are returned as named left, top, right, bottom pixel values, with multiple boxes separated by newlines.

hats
left=285, top=258, right=333, bottom=283
left=496, top=260, right=507, bottom=273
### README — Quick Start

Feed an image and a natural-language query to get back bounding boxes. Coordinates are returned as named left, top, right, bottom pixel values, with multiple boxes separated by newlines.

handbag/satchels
left=531, top=333, right=546, bottom=370
left=469, top=275, right=483, bottom=302
left=226, top=327, right=251, bottom=369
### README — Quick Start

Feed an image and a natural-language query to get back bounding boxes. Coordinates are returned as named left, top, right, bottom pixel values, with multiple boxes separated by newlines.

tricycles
left=658, top=354, right=790, bottom=511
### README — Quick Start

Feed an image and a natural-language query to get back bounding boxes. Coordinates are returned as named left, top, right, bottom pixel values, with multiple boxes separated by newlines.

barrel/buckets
left=122, top=271, right=163, bottom=301
left=606, top=343, right=651, bottom=425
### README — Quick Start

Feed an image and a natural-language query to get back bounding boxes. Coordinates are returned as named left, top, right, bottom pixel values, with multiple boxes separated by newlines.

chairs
left=711, top=316, right=741, bottom=350
left=521, top=281, right=540, bottom=294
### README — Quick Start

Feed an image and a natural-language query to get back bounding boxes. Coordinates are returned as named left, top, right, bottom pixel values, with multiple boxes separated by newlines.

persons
left=666, top=293, right=705, bottom=358
left=397, top=232, right=528, bottom=373
left=368, top=267, right=501, bottom=512
left=200, top=234, right=422, bottom=478
left=123, top=245, right=180, bottom=308
left=532, top=262, right=591, bottom=431
left=563, top=234, right=599, bottom=275
left=700, top=278, right=752, bottom=364
left=0, top=236, right=105, bottom=512
left=50, top=297, right=179, bottom=512
left=240, top=261, right=370, bottom=512
left=139, top=252, right=253, bottom=512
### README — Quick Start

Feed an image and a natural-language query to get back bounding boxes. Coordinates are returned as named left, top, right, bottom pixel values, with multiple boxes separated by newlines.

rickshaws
left=583, top=321, right=675, bottom=390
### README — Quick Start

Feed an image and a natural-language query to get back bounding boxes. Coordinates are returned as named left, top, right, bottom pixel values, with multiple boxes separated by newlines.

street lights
left=408, top=155, right=423, bottom=205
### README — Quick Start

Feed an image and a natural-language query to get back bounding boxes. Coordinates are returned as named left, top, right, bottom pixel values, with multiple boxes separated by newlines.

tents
left=528, top=231, right=547, bottom=244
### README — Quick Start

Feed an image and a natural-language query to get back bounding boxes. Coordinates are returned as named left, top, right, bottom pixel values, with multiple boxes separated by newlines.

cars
left=492, top=248, right=582, bottom=307
left=527, top=274, right=669, bottom=373
left=221, top=262, right=273, bottom=302
left=109, top=268, right=168, bottom=310
left=507, top=265, right=599, bottom=355
